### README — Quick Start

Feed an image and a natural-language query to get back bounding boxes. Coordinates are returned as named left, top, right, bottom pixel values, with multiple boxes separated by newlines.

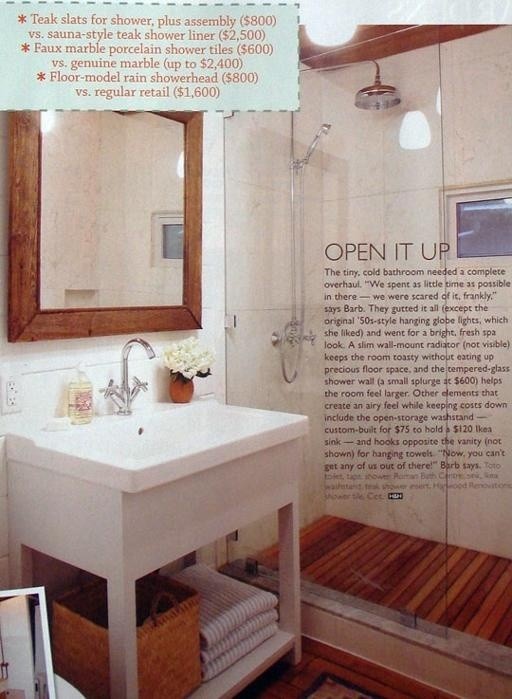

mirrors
left=8, top=110, right=204, bottom=343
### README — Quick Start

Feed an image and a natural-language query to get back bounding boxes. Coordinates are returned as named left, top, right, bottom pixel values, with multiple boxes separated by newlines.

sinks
left=4, top=341, right=308, bottom=493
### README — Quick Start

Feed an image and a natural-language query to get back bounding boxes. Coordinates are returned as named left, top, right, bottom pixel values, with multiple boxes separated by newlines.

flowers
left=164, top=336, right=215, bottom=384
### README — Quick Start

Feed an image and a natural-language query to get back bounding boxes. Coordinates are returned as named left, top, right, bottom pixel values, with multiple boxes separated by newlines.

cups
left=44, top=405, right=68, bottom=431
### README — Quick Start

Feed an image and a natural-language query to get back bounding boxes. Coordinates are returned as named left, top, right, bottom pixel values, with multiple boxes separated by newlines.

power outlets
left=0, top=367, right=24, bottom=414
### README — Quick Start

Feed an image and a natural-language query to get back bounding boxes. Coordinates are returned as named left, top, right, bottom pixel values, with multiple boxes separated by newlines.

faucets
left=98, top=337, right=155, bottom=416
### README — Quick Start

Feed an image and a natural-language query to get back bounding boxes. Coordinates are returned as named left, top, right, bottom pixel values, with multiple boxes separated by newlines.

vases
left=170, top=377, right=194, bottom=403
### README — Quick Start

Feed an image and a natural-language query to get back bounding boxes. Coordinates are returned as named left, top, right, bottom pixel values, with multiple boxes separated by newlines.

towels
left=202, top=608, right=278, bottom=667
left=202, top=624, right=278, bottom=680
left=169, top=562, right=278, bottom=651
